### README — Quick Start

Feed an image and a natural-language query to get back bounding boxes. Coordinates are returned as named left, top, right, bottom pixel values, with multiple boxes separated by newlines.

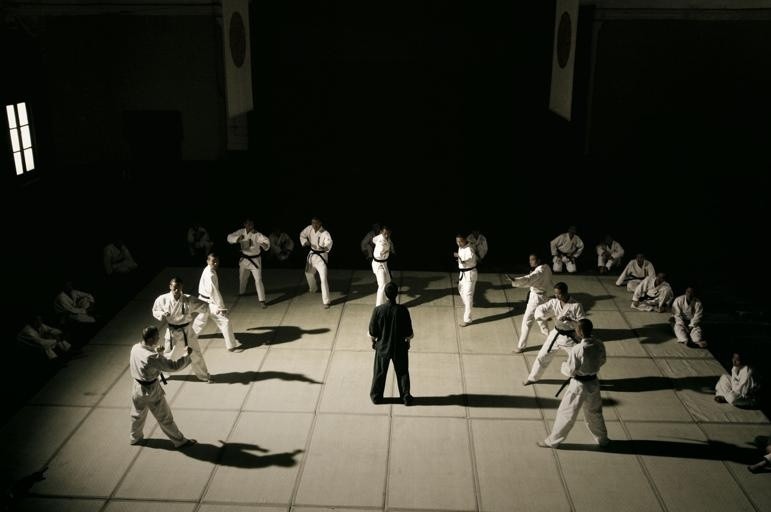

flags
left=221, top=0, right=255, bottom=119
left=547, top=2, right=580, bottom=122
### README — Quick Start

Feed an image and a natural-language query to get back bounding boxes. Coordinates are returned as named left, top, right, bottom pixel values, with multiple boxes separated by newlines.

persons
left=596, top=236, right=673, bottom=312
left=127, top=326, right=196, bottom=451
left=150, top=278, right=214, bottom=383
left=188, top=222, right=209, bottom=249
left=17, top=237, right=140, bottom=366
left=521, top=281, right=585, bottom=385
left=551, top=226, right=584, bottom=273
left=300, top=219, right=333, bottom=309
left=369, top=282, right=417, bottom=406
left=715, top=351, right=763, bottom=406
left=504, top=254, right=553, bottom=353
left=534, top=319, right=610, bottom=449
left=190, top=252, right=246, bottom=352
left=672, top=286, right=703, bottom=345
left=454, top=228, right=487, bottom=326
left=361, top=224, right=395, bottom=307
left=226, top=218, right=293, bottom=308
left=748, top=446, right=771, bottom=470
